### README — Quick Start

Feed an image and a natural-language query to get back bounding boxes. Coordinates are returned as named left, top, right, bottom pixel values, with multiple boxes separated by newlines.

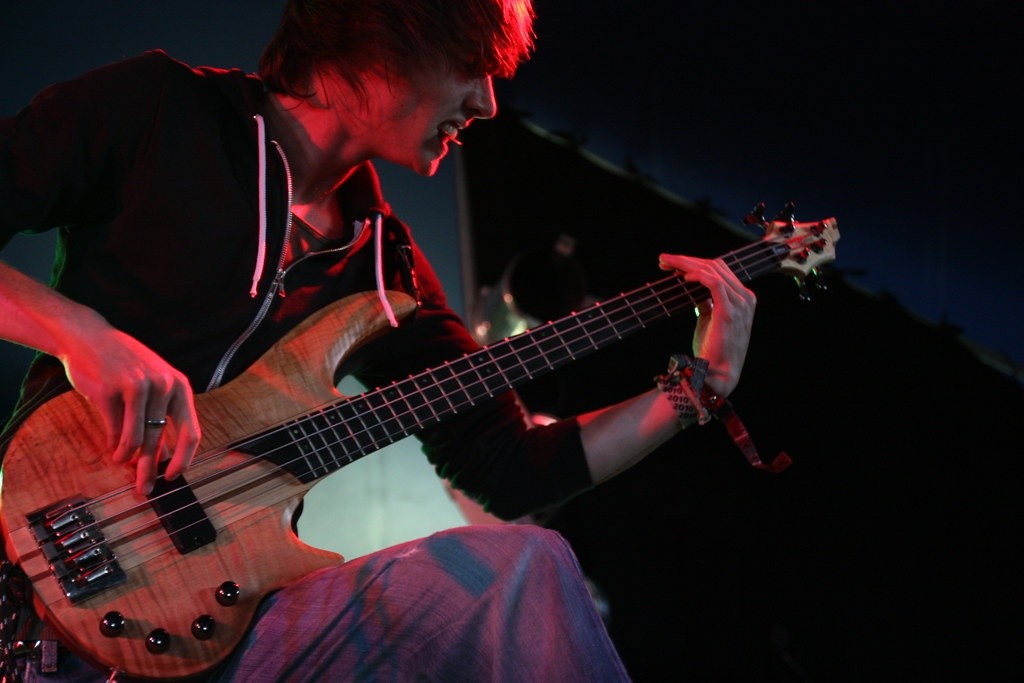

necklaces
left=290, top=210, right=350, bottom=248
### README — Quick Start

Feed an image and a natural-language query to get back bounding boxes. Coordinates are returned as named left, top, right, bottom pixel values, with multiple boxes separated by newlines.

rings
left=143, top=417, right=166, bottom=429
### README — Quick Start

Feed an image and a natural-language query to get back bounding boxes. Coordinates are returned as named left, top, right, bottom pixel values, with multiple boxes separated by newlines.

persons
left=0, top=0, right=755, bottom=683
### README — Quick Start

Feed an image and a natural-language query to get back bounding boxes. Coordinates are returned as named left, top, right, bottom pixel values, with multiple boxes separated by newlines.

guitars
left=0, top=203, right=842, bottom=680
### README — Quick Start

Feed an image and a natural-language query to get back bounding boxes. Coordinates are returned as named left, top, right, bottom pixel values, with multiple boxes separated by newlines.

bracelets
left=656, top=352, right=796, bottom=474
